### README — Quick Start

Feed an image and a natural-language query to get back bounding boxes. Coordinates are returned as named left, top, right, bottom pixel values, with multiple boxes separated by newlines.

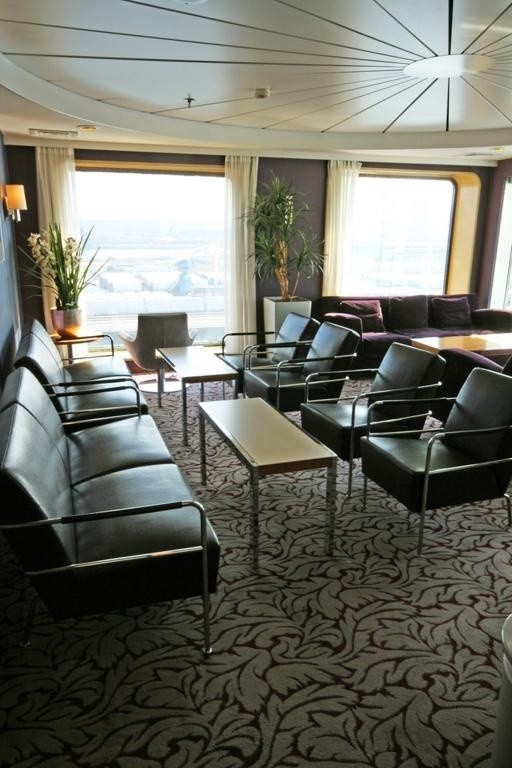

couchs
left=311, top=295, right=512, bottom=372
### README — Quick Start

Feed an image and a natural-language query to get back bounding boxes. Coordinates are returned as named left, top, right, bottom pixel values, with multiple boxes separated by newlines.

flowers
left=15, top=224, right=111, bottom=309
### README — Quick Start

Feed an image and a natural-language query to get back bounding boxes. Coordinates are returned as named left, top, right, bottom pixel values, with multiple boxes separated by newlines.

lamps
left=2, top=178, right=29, bottom=223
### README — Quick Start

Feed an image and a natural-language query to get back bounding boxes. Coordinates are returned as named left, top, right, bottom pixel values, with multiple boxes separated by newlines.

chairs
left=116, top=311, right=196, bottom=392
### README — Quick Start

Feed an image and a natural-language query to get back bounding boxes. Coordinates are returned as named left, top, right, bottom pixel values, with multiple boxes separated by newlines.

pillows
left=338, top=295, right=472, bottom=332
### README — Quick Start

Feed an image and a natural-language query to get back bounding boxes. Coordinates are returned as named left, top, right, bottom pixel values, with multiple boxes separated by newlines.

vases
left=50, top=307, right=82, bottom=337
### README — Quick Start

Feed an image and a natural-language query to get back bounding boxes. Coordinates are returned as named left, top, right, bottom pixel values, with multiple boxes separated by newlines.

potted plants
left=236, top=169, right=330, bottom=360
